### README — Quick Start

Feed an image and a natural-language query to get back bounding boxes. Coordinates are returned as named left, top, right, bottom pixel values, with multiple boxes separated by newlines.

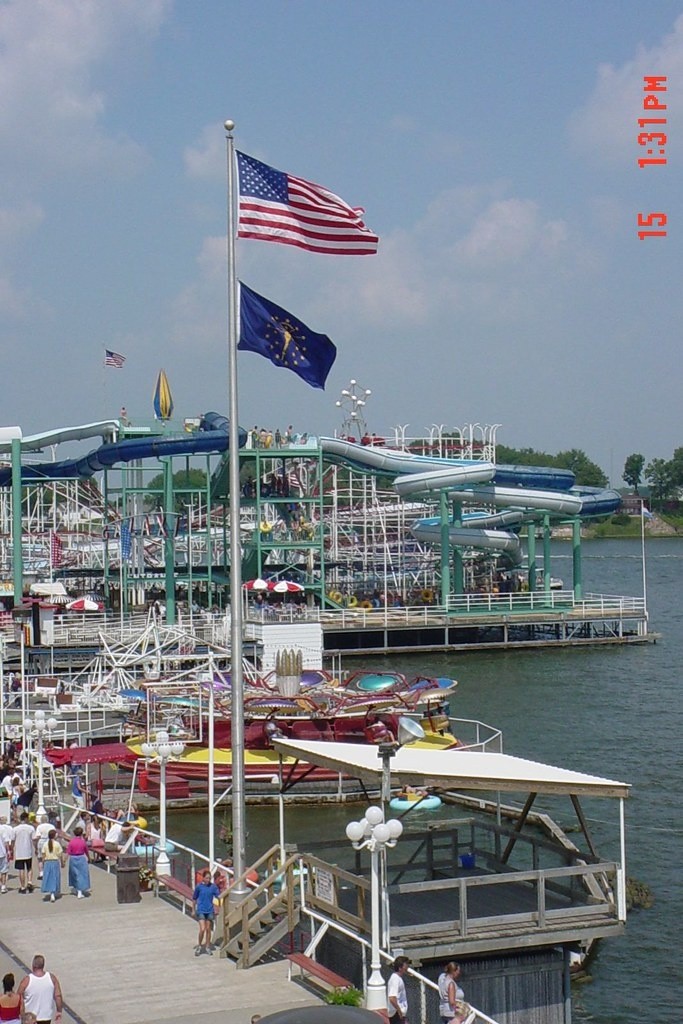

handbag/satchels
left=91, top=838, right=105, bottom=847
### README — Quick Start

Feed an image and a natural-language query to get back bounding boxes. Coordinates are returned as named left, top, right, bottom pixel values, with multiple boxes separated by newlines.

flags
left=105, top=349, right=126, bottom=369
left=235, top=147, right=379, bottom=256
left=237, top=280, right=337, bottom=392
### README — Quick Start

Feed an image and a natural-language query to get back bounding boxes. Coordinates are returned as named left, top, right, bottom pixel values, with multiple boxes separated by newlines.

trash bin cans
left=116, top=854, right=142, bottom=904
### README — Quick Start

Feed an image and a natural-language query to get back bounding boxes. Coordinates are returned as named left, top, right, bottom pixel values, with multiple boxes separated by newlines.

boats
left=45, top=605, right=506, bottom=816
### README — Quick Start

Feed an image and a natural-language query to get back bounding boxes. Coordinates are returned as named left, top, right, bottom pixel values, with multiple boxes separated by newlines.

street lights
left=344, top=805, right=406, bottom=1015
left=21, top=710, right=57, bottom=823
left=141, top=731, right=184, bottom=888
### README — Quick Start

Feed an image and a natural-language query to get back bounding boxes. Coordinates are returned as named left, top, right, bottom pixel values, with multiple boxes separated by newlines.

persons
left=265, top=715, right=289, bottom=741
left=339, top=433, right=346, bottom=439
left=252, top=590, right=264, bottom=620
left=252, top=424, right=294, bottom=449
left=0, top=709, right=150, bottom=903
left=0, top=955, right=63, bottom=1024
left=246, top=472, right=289, bottom=497
left=364, top=432, right=377, bottom=437
left=11, top=676, right=21, bottom=708
left=120, top=406, right=127, bottom=425
left=386, top=956, right=469, bottom=1024
left=192, top=600, right=200, bottom=619
left=153, top=600, right=166, bottom=621
left=192, top=870, right=220, bottom=956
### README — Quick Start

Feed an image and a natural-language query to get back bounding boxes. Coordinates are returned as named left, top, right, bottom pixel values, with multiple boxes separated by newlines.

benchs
left=155, top=874, right=219, bottom=932
left=284, top=954, right=351, bottom=990
left=57, top=829, right=119, bottom=873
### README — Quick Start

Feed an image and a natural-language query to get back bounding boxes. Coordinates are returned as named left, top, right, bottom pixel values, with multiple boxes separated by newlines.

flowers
left=139, top=866, right=154, bottom=883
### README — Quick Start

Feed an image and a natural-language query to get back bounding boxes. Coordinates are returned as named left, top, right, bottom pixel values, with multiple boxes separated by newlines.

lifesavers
left=421, top=589, right=433, bottom=602
left=329, top=589, right=380, bottom=610
left=520, top=582, right=529, bottom=592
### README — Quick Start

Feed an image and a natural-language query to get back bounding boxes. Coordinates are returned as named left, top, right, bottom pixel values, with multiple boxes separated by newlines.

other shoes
left=27, top=883, right=33, bottom=891
left=19, top=887, right=26, bottom=893
left=1, top=888, right=8, bottom=893
left=205, top=946, right=213, bottom=955
left=37, top=876, right=43, bottom=879
left=195, top=946, right=203, bottom=956
left=50, top=895, right=55, bottom=902
left=78, top=894, right=84, bottom=899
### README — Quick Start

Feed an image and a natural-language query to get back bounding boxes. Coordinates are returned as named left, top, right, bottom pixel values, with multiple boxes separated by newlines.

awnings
left=0, top=582, right=68, bottom=596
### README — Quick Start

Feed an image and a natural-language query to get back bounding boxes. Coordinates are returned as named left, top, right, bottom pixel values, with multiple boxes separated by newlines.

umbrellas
left=242, top=577, right=305, bottom=604
left=44, top=593, right=107, bottom=613
left=152, top=368, right=175, bottom=426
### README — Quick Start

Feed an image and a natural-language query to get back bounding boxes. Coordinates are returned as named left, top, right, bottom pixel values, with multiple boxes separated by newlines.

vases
left=139, top=879, right=149, bottom=891
